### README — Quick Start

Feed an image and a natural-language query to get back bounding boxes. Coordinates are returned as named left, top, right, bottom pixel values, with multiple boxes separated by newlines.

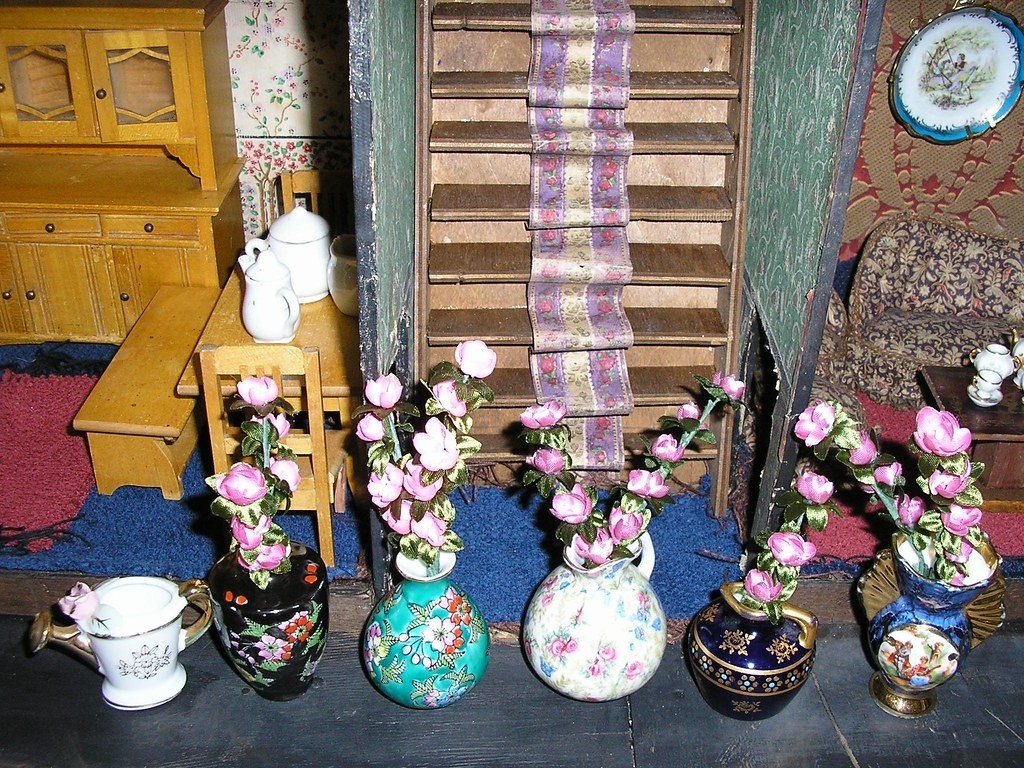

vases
left=209, top=539, right=330, bottom=702
left=688, top=583, right=820, bottom=721
left=523, top=527, right=668, bottom=704
left=867, top=531, right=1000, bottom=718
left=363, top=552, right=491, bottom=711
left=30, top=573, right=215, bottom=712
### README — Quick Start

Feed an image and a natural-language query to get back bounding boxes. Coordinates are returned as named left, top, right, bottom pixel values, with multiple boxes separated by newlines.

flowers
left=353, top=337, right=499, bottom=579
left=736, top=395, right=857, bottom=626
left=836, top=401, right=987, bottom=591
left=59, top=582, right=110, bottom=640
left=207, top=372, right=302, bottom=586
left=516, top=358, right=749, bottom=565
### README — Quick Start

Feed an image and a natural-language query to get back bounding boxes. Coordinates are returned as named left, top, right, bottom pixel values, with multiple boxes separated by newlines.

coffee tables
left=914, top=363, right=1024, bottom=490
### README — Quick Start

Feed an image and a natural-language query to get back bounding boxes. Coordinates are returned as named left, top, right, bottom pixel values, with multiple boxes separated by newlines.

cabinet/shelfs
left=0, top=0, right=245, bottom=344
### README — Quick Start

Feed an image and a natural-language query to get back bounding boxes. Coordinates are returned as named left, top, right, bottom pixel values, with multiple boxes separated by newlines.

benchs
left=72, top=282, right=223, bottom=500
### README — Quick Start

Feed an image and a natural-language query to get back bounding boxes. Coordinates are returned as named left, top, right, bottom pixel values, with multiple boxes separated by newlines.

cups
left=325, top=236, right=359, bottom=320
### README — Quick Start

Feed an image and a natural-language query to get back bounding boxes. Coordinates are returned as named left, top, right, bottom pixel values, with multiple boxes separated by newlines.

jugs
left=29, top=575, right=215, bottom=710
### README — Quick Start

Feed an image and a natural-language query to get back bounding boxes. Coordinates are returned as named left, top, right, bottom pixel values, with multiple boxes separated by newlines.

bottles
left=969, top=344, right=1021, bottom=381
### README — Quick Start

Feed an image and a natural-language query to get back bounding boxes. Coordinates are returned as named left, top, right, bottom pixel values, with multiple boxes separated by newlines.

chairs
left=200, top=342, right=337, bottom=567
left=280, top=168, right=357, bottom=237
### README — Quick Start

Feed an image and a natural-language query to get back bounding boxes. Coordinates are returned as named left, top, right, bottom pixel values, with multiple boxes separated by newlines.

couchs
left=736, top=211, right=1024, bottom=481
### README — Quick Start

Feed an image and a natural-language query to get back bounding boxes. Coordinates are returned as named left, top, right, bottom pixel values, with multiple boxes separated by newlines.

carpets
left=804, top=387, right=1024, bottom=564
left=0, top=368, right=99, bottom=551
left=964, top=452, right=1024, bottom=514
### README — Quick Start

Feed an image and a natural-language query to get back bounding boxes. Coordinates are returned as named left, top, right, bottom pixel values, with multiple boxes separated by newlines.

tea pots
left=245, top=206, right=331, bottom=307
left=1011, top=327, right=1024, bottom=362
left=238, top=251, right=300, bottom=346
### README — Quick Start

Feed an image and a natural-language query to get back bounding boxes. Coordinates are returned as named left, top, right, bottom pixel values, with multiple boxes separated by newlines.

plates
left=966, top=384, right=1003, bottom=408
left=888, top=6, right=1024, bottom=147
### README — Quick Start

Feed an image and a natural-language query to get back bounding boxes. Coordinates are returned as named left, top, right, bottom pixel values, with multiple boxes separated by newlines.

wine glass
left=1013, top=365, right=1024, bottom=404
left=973, top=368, right=1003, bottom=400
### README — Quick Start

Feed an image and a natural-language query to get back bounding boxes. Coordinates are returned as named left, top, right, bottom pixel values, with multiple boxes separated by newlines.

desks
left=173, top=264, right=363, bottom=510
